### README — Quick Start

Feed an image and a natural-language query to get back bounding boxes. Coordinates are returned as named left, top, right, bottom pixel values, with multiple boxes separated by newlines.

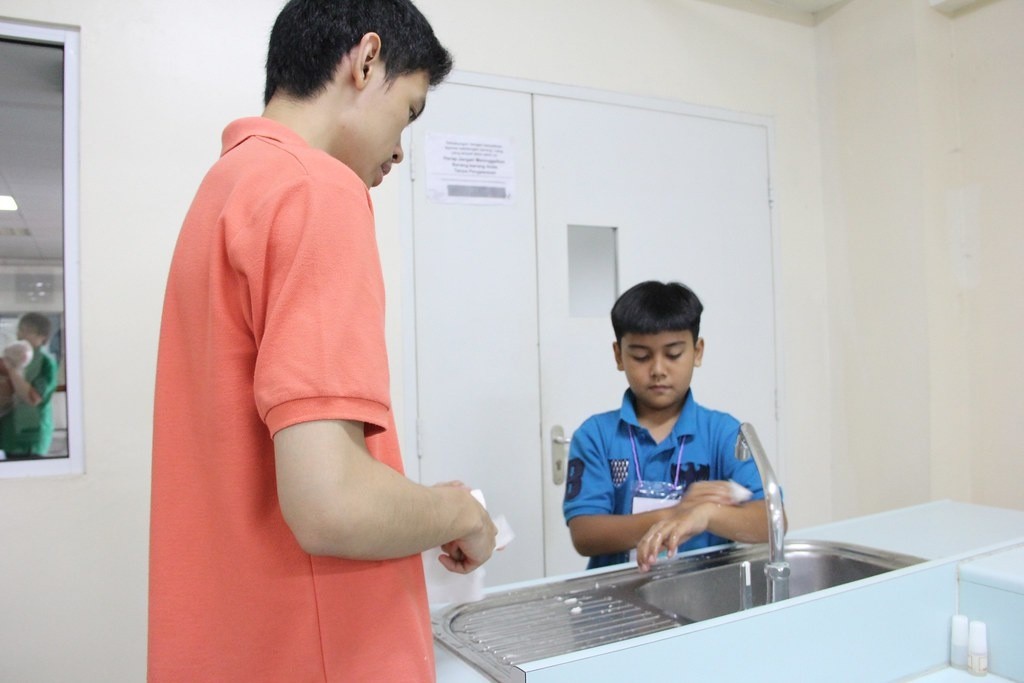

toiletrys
left=951, top=613, right=989, bottom=678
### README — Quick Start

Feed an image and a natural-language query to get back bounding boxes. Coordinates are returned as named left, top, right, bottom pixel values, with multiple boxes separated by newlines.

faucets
left=733, top=421, right=790, bottom=606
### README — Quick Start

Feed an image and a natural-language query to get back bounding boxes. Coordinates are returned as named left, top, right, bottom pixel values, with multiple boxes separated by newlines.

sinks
left=609, top=537, right=931, bottom=622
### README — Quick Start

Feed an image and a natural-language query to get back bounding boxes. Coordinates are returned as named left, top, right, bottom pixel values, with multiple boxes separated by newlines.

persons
left=560, top=280, right=787, bottom=571
left=147, top=1, right=498, bottom=683
left=0, top=312, right=58, bottom=458
